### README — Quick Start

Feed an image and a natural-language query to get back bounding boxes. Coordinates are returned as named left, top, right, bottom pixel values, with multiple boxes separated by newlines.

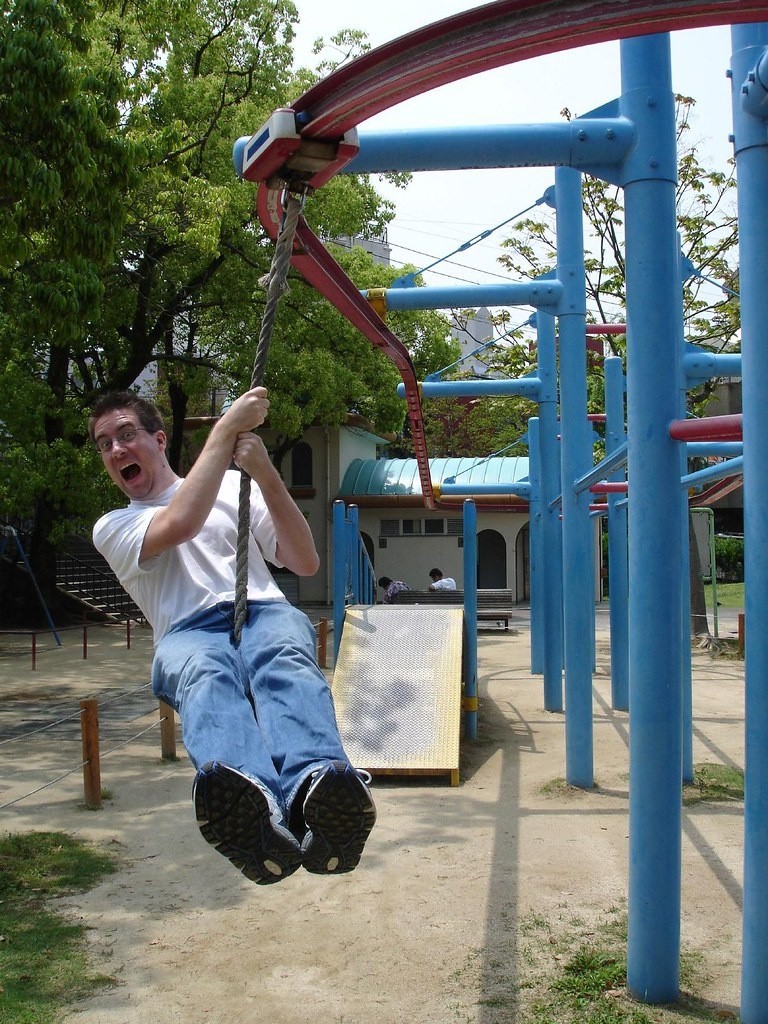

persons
left=377, top=576, right=413, bottom=605
left=87, top=386, right=378, bottom=887
left=428, top=568, right=457, bottom=591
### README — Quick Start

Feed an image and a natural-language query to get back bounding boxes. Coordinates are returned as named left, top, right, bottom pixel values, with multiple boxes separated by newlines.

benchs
left=392, top=588, right=513, bottom=631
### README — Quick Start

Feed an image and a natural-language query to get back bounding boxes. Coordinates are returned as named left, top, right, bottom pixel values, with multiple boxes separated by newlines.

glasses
left=95, top=428, right=148, bottom=454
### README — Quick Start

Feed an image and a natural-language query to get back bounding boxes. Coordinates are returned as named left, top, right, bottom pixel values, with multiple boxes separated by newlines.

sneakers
left=192, top=761, right=301, bottom=886
left=302, top=761, right=377, bottom=875
